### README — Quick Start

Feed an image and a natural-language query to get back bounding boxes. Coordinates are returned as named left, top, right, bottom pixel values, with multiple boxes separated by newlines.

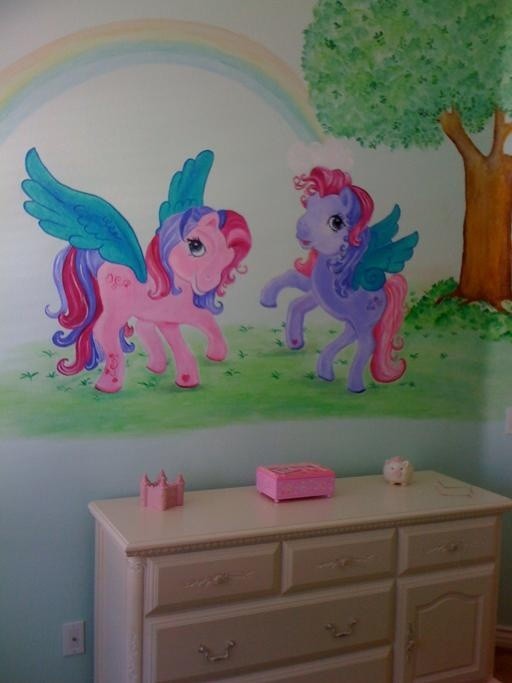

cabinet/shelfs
left=87, top=470, right=512, bottom=683
